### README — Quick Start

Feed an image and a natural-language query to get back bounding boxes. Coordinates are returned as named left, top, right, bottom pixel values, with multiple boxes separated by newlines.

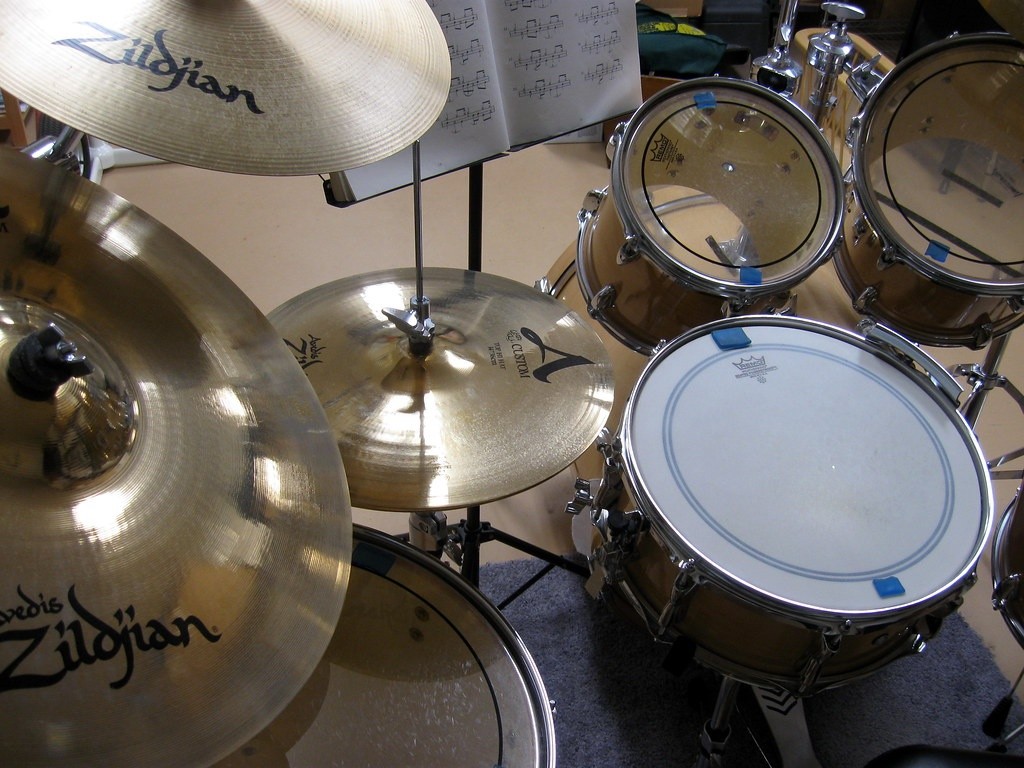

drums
left=220, top=523, right=559, bottom=768
left=573, top=74, right=846, bottom=358
left=596, top=311, right=998, bottom=693
left=990, top=479, right=1024, bottom=648
left=833, top=31, right=1024, bottom=346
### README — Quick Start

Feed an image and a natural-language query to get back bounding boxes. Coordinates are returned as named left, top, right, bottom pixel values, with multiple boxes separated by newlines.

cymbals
left=0, top=145, right=354, bottom=768
left=263, top=265, right=617, bottom=514
left=0, top=0, right=452, bottom=178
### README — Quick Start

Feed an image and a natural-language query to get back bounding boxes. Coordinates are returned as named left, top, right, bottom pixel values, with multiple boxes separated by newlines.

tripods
left=945, top=330, right=1024, bottom=437
left=323, top=107, right=641, bottom=611
